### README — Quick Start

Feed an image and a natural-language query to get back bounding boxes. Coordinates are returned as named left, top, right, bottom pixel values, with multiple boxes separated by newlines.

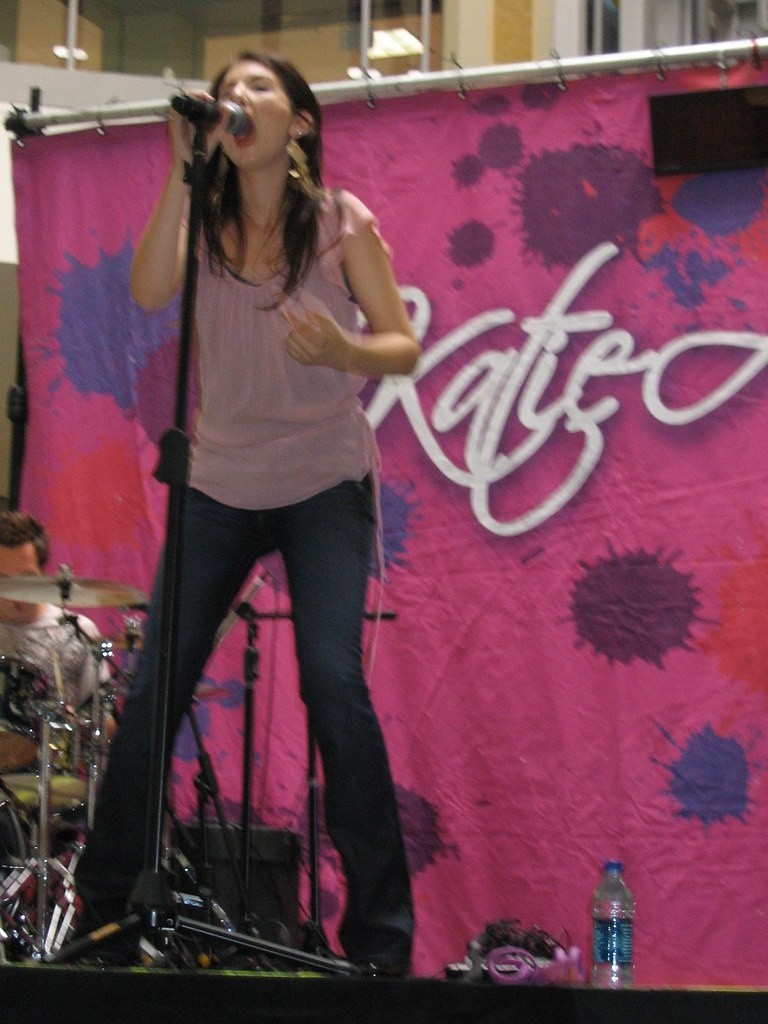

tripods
left=0, top=603, right=379, bottom=980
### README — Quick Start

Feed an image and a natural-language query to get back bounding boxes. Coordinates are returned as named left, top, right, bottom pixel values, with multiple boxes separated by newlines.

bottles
left=592, top=859, right=635, bottom=989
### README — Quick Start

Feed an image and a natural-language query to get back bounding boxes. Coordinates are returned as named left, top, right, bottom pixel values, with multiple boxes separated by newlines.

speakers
left=173, top=820, right=303, bottom=974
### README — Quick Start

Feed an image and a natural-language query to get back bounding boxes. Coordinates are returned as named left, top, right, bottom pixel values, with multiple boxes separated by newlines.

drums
left=0, top=699, right=94, bottom=812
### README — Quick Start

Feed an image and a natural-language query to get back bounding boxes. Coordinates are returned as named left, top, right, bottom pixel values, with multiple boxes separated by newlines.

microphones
left=212, top=573, right=269, bottom=648
left=172, top=96, right=247, bottom=135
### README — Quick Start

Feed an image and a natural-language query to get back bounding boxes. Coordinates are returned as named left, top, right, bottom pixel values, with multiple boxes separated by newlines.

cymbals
left=87, top=632, right=144, bottom=651
left=1, top=573, right=145, bottom=607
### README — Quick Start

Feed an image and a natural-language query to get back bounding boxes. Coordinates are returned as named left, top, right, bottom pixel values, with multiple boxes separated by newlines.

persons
left=0, top=514, right=115, bottom=771
left=72, top=51, right=421, bottom=976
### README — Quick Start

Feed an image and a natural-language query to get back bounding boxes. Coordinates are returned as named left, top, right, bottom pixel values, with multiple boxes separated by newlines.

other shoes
left=64, top=935, right=143, bottom=967
left=356, top=946, right=413, bottom=978
